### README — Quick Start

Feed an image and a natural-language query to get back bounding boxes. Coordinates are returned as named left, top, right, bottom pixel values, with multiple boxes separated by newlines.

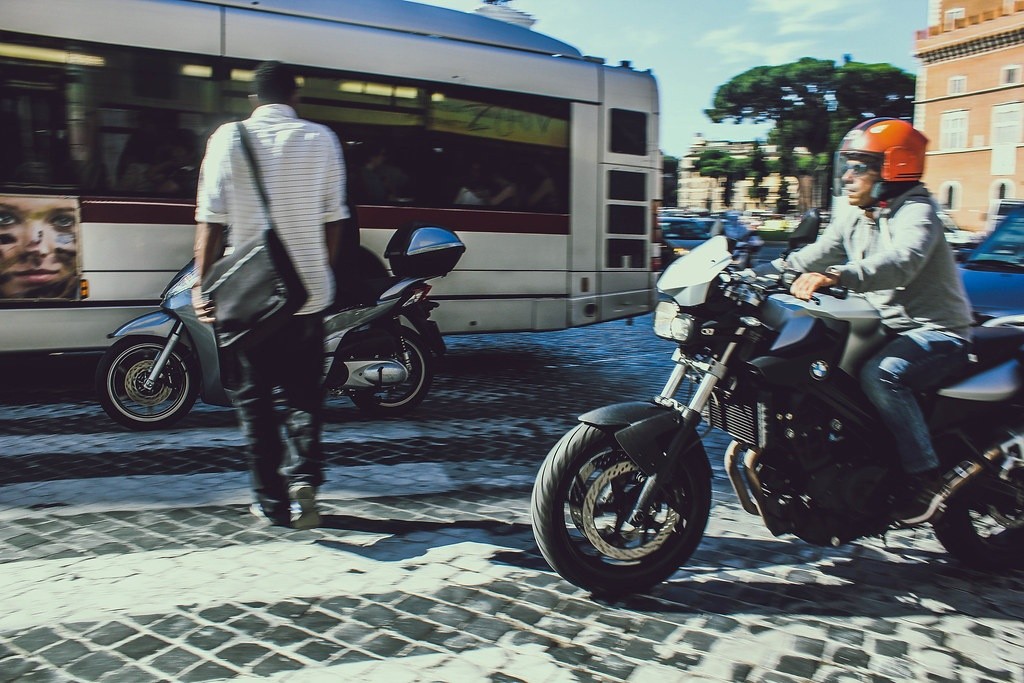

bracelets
left=829, top=268, right=841, bottom=274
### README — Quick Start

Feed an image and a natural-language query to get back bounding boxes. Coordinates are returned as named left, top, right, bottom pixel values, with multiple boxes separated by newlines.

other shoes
left=288, top=482, right=323, bottom=528
left=897, top=465, right=945, bottom=524
left=250, top=502, right=276, bottom=526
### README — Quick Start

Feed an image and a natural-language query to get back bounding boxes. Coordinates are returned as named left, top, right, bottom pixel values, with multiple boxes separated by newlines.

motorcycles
left=92, top=221, right=468, bottom=435
left=527, top=221, right=1023, bottom=596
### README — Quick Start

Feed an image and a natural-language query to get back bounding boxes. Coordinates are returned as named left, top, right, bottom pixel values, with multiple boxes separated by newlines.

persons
left=724, top=210, right=751, bottom=254
left=0, top=194, right=81, bottom=301
left=738, top=116, right=975, bottom=524
left=189, top=62, right=352, bottom=530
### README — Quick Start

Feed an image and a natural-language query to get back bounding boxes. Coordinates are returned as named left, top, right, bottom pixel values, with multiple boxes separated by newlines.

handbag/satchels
left=201, top=229, right=309, bottom=352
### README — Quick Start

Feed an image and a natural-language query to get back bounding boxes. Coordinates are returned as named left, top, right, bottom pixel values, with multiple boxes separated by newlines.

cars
left=656, top=206, right=765, bottom=278
left=935, top=198, right=1024, bottom=323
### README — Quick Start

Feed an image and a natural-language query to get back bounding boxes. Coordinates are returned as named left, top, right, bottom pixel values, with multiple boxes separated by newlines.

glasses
left=840, top=163, right=882, bottom=177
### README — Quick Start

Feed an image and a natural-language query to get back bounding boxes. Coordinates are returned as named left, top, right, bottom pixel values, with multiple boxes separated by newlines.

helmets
left=832, top=117, right=928, bottom=182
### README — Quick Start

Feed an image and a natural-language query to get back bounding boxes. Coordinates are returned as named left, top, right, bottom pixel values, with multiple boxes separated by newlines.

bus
left=0, top=0, right=660, bottom=360
